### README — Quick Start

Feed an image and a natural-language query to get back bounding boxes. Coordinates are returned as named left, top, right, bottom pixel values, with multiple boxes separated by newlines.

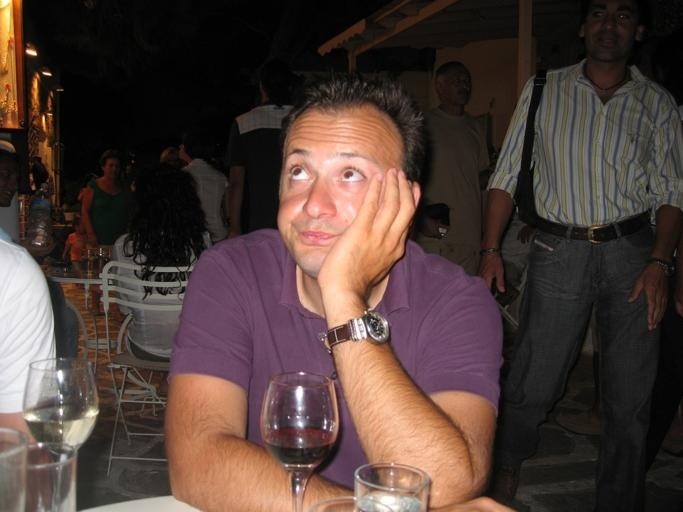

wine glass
left=77, top=248, right=98, bottom=313
left=259, top=371, right=340, bottom=512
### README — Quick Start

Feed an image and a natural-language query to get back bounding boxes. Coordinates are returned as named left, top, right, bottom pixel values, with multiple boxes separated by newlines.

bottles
left=27, top=182, right=52, bottom=249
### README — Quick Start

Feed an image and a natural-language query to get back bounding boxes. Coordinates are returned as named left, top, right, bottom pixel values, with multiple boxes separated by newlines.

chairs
left=97, top=263, right=196, bottom=473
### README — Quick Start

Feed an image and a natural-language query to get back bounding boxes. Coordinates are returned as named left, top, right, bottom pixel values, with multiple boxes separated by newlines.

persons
left=164, top=78, right=516, bottom=512
left=2, top=238, right=58, bottom=418
left=411, top=61, right=490, bottom=277
left=78, top=62, right=299, bottom=393
left=476, top=3, right=681, bottom=512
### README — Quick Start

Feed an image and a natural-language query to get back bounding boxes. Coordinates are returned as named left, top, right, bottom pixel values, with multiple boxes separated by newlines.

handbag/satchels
left=493, top=219, right=531, bottom=303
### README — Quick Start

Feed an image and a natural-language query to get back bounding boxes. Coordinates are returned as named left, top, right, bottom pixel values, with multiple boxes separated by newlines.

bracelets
left=481, top=248, right=500, bottom=254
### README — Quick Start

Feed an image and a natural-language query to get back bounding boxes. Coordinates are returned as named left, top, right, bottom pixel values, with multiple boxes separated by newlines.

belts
left=532, top=208, right=652, bottom=241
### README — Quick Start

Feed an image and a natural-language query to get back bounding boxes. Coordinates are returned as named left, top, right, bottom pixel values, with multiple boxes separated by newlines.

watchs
left=647, top=257, right=674, bottom=276
left=326, top=311, right=390, bottom=350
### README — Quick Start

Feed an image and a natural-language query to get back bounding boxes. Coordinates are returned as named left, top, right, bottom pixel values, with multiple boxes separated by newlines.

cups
left=310, top=497, right=392, bottom=511
left=22, top=359, right=101, bottom=458
left=1, top=428, right=26, bottom=511
left=95, top=246, right=117, bottom=280
left=353, top=461, right=432, bottom=512
left=1, top=442, right=77, bottom=512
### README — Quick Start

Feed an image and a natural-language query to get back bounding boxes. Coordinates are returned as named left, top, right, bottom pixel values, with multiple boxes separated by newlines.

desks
left=50, top=263, right=117, bottom=345
